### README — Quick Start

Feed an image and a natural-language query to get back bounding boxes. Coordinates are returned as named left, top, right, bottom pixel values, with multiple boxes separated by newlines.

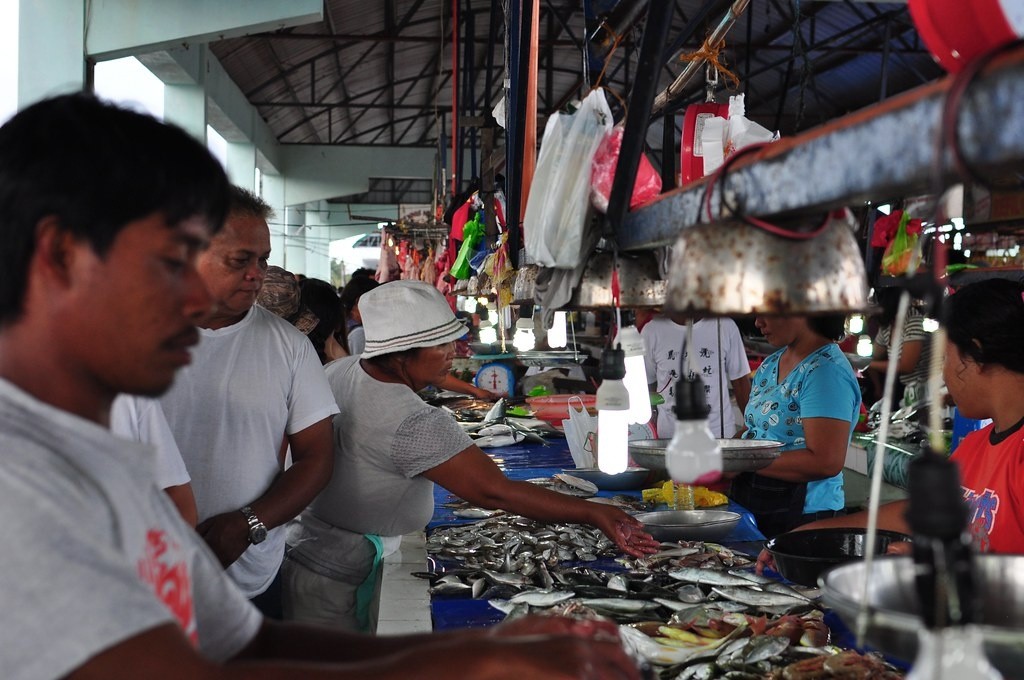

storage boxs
left=949, top=407, right=993, bottom=454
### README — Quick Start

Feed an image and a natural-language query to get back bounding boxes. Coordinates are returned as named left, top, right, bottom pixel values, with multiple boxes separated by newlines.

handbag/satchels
left=523, top=88, right=662, bottom=269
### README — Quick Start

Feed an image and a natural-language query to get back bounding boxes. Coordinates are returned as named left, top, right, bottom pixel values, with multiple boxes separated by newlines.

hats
left=357, top=280, right=470, bottom=359
left=254, top=266, right=320, bottom=335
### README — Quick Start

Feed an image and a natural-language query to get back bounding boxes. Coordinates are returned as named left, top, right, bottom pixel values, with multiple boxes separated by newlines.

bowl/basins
left=564, top=466, right=649, bottom=490
left=632, top=509, right=742, bottom=542
left=764, top=526, right=913, bottom=587
left=626, top=437, right=785, bottom=472
left=525, top=393, right=596, bottom=426
left=818, top=552, right=1024, bottom=680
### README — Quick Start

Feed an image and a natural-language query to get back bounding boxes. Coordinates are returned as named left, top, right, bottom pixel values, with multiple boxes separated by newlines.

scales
left=468, top=342, right=519, bottom=402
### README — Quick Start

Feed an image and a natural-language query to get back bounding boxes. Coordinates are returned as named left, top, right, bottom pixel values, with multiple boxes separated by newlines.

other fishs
left=412, top=473, right=901, bottom=680
left=440, top=397, right=566, bottom=449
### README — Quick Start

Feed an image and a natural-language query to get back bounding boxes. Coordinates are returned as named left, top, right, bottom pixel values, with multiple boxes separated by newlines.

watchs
left=239, top=505, right=267, bottom=546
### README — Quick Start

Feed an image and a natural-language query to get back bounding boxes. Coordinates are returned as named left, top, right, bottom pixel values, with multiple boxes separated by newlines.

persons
left=528, top=308, right=618, bottom=396
left=754, top=275, right=1023, bottom=583
left=653, top=312, right=864, bottom=541
left=108, top=389, right=197, bottom=528
left=0, top=91, right=637, bottom=680
left=944, top=247, right=970, bottom=295
left=281, top=278, right=660, bottom=640
left=256, top=267, right=498, bottom=404
left=860, top=286, right=931, bottom=412
left=157, top=185, right=340, bottom=618
left=641, top=307, right=751, bottom=441
